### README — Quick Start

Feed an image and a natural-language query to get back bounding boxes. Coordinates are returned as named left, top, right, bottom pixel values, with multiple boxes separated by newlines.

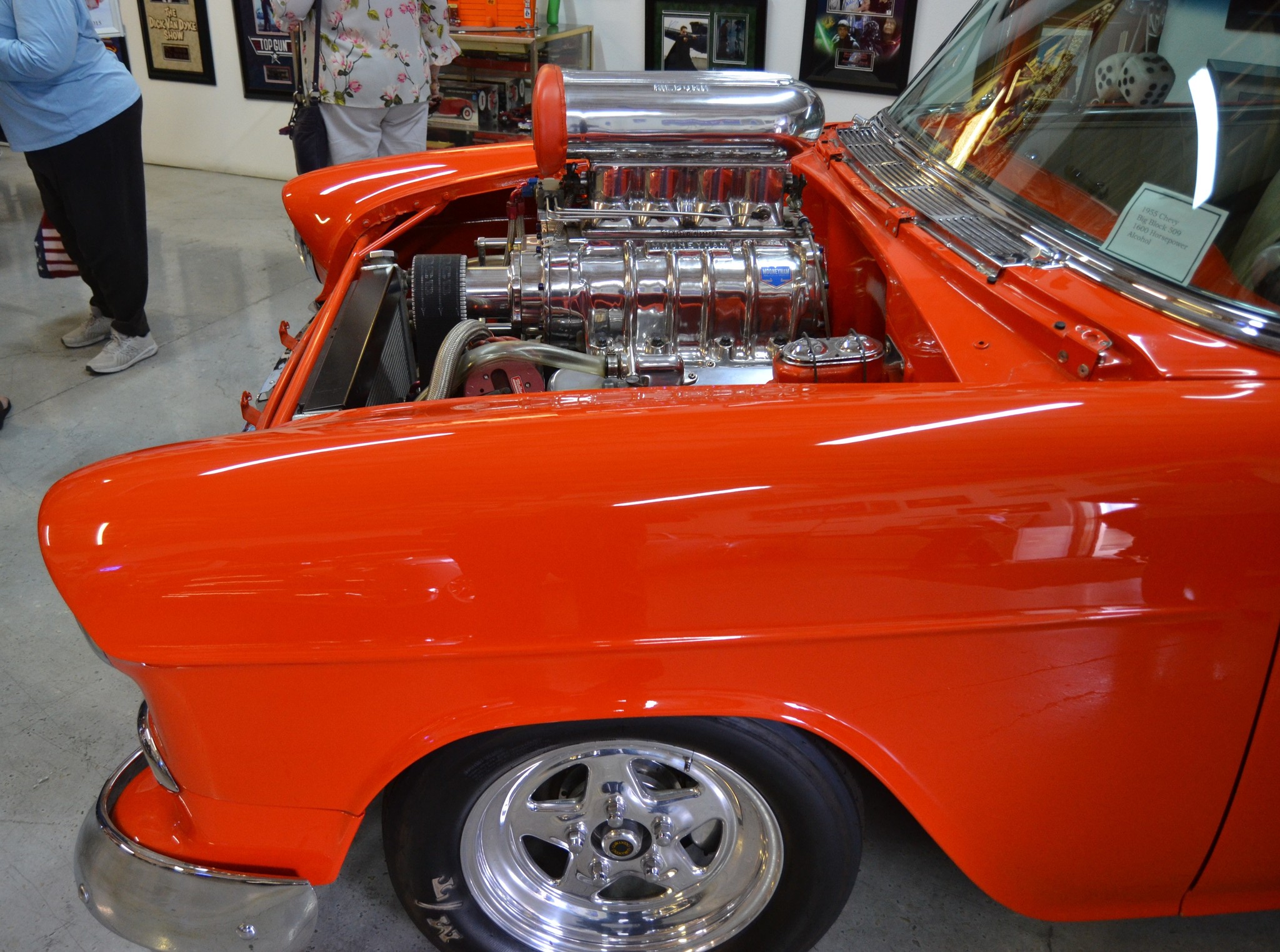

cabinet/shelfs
left=424, top=23, right=593, bottom=146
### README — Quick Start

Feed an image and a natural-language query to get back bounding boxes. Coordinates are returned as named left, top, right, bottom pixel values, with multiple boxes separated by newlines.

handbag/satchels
left=34, top=210, right=79, bottom=279
left=287, top=94, right=327, bottom=175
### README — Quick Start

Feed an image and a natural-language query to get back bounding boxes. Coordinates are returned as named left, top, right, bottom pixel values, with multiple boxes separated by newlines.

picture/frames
left=85, top=0, right=126, bottom=38
left=1205, top=58, right=1280, bottom=113
left=230, top=0, right=300, bottom=102
left=798, top=0, right=917, bottom=98
left=137, top=0, right=218, bottom=86
left=100, top=37, right=131, bottom=75
left=642, top=0, right=767, bottom=74
left=1223, top=0, right=1280, bottom=37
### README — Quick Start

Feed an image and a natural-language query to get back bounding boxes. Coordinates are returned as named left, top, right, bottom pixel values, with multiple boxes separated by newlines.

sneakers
left=85, top=326, right=157, bottom=373
left=61, top=297, right=112, bottom=347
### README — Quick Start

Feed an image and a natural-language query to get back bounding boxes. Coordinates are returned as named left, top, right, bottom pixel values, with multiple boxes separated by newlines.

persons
left=814, top=0, right=903, bottom=71
left=0, top=1, right=160, bottom=375
left=734, top=20, right=745, bottom=59
left=664, top=25, right=698, bottom=71
left=267, top=0, right=463, bottom=167
left=718, top=19, right=730, bottom=58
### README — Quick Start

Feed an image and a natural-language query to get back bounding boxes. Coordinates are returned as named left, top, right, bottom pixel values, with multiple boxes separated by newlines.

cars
left=38, top=0, right=1280, bottom=950
